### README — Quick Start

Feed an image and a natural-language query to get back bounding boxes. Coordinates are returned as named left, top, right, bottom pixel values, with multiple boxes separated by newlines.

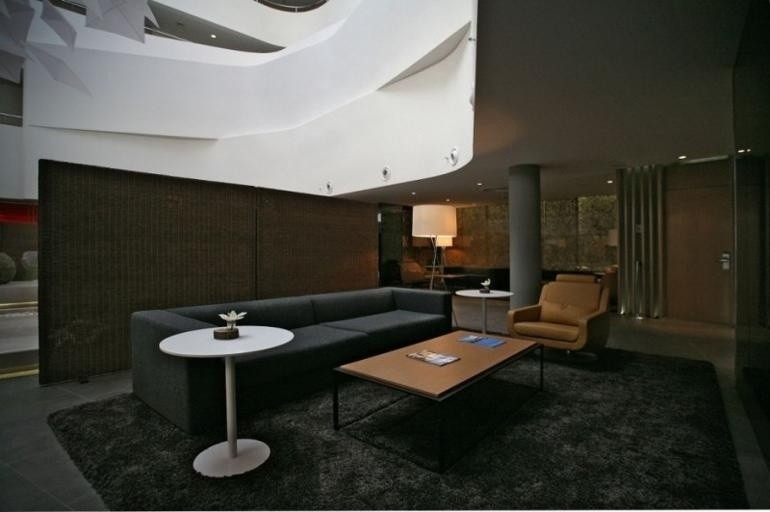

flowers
left=218, top=310, right=247, bottom=330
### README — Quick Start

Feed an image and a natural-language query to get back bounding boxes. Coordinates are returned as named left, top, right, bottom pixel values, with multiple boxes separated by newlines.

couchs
left=131, top=285, right=453, bottom=433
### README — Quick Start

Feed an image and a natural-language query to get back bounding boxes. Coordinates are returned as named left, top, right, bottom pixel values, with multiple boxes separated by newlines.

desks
left=455, top=289, right=514, bottom=336
left=159, top=326, right=295, bottom=478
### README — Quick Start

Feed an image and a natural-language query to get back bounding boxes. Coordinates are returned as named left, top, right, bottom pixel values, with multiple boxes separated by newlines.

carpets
left=47, top=325, right=751, bottom=510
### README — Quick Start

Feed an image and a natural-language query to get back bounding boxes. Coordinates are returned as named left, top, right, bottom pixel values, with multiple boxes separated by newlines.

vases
left=214, top=321, right=238, bottom=338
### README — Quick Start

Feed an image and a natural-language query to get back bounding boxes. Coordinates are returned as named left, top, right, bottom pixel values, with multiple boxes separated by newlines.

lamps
left=411, top=204, right=458, bottom=290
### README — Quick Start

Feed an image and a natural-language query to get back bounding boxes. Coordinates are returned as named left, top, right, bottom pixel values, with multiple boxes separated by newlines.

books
left=406, top=348, right=460, bottom=367
left=457, top=334, right=506, bottom=349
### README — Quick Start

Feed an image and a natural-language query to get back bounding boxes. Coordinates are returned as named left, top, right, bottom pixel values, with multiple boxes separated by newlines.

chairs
left=505, top=273, right=610, bottom=367
